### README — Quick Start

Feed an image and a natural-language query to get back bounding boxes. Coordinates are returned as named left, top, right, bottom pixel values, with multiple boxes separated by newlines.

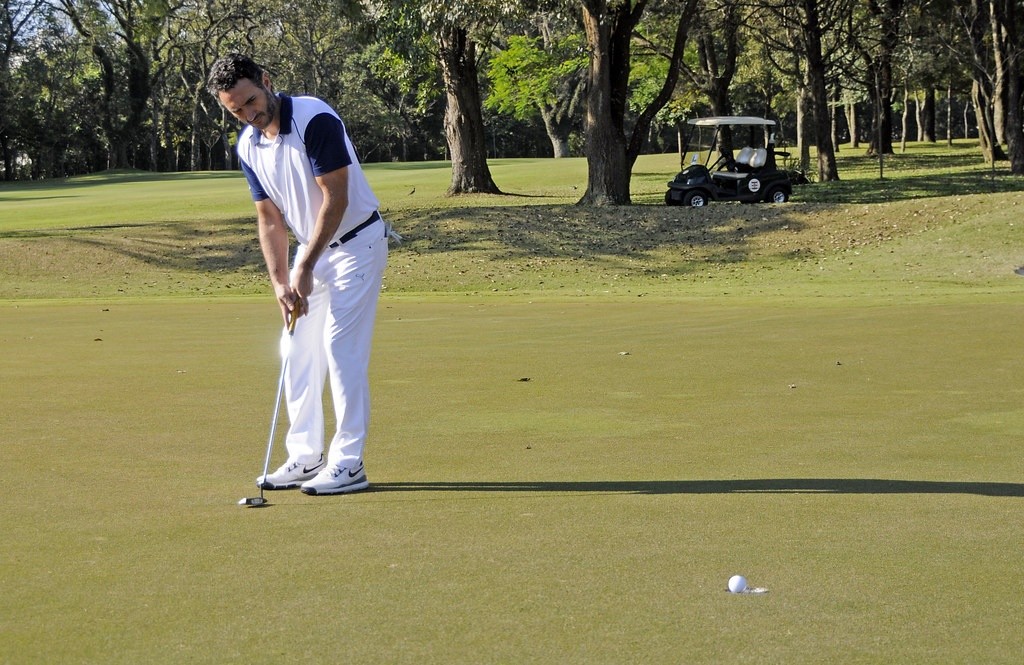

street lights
left=873, top=56, right=883, bottom=179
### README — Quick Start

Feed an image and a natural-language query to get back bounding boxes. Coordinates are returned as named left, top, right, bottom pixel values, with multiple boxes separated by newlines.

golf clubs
left=238, top=299, right=299, bottom=506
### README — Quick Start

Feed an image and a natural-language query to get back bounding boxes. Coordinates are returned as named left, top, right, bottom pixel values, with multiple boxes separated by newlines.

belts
left=328, top=210, right=380, bottom=249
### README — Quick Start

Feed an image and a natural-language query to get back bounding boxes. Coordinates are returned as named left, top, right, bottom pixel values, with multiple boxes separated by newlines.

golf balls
left=728, top=574, right=748, bottom=594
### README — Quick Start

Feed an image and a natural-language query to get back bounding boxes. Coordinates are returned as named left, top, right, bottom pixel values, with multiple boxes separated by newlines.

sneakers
left=255, top=458, right=326, bottom=491
left=301, top=461, right=370, bottom=495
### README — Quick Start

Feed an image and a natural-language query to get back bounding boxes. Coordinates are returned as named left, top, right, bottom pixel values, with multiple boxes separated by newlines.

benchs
left=713, top=146, right=767, bottom=189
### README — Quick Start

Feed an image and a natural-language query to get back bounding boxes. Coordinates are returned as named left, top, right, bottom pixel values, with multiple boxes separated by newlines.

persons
left=206, top=53, right=388, bottom=496
